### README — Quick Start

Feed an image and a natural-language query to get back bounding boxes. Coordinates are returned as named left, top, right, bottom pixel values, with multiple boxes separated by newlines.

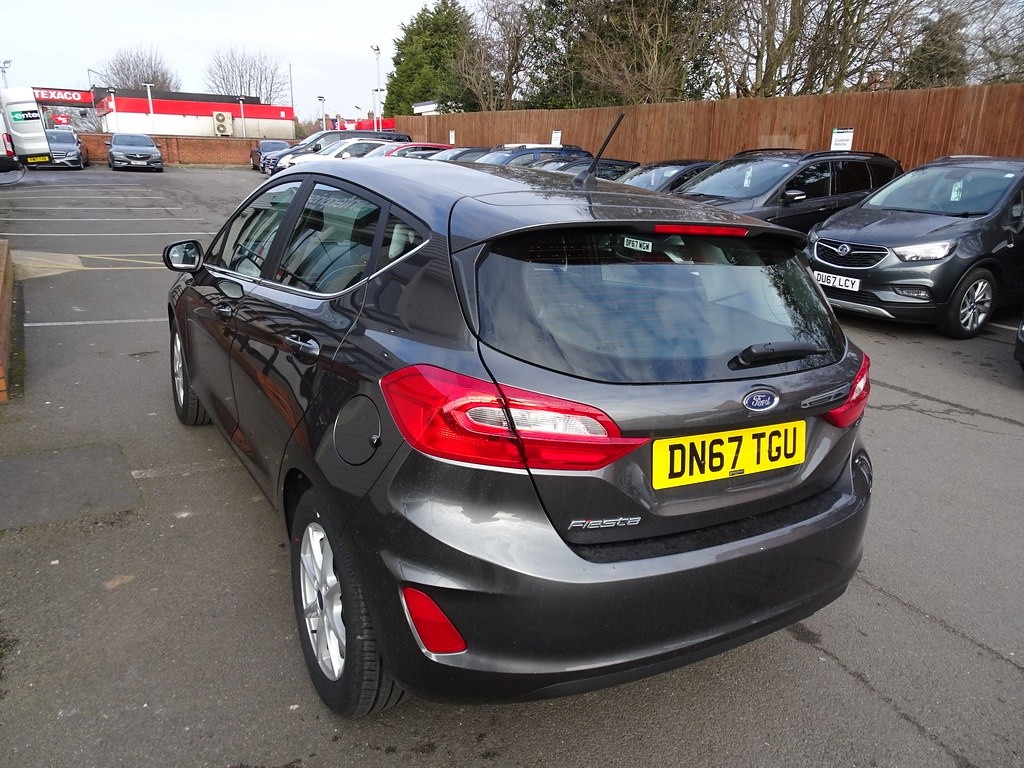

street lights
left=371, top=44, right=383, bottom=134
left=141, top=83, right=155, bottom=114
left=317, top=95, right=327, bottom=132
left=236, top=96, right=249, bottom=139
left=373, top=88, right=385, bottom=133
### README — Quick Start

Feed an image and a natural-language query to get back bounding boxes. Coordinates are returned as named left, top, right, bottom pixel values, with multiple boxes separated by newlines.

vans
left=0, top=86, right=54, bottom=174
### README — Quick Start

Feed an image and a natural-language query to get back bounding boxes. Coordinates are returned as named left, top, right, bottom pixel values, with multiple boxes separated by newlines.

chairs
left=324, top=205, right=378, bottom=297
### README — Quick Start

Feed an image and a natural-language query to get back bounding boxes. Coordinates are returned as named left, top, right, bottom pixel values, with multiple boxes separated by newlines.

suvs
left=474, top=141, right=597, bottom=175
left=161, top=152, right=883, bottom=720
left=663, top=147, right=907, bottom=234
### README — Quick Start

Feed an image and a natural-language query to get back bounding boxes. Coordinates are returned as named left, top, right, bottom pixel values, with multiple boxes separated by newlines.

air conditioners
left=215, top=123, right=234, bottom=135
left=213, top=111, right=232, bottom=123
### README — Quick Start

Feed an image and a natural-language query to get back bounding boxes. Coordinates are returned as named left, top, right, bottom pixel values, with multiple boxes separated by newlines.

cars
left=801, top=155, right=1024, bottom=340
left=250, top=140, right=292, bottom=171
left=257, top=130, right=492, bottom=180
left=105, top=133, right=163, bottom=173
left=527, top=159, right=639, bottom=184
left=28, top=128, right=90, bottom=171
left=610, top=159, right=715, bottom=193
